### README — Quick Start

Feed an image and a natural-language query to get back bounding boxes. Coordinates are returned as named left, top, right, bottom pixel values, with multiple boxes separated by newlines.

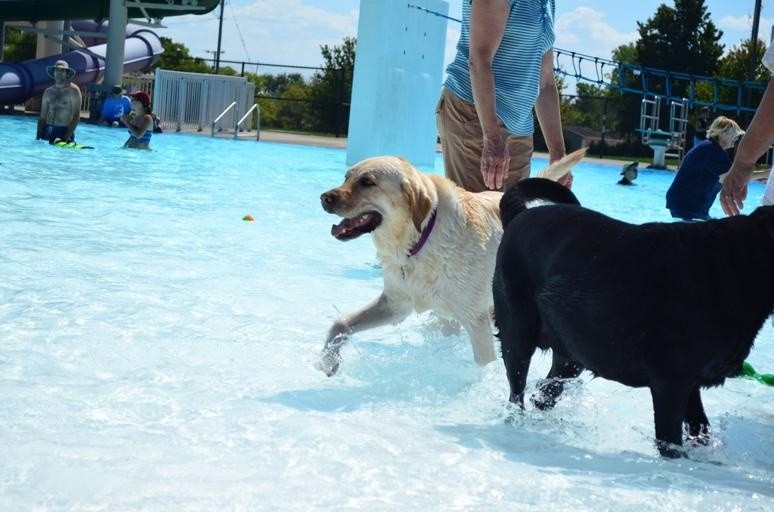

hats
left=128, top=90, right=151, bottom=104
left=109, top=85, right=126, bottom=95
left=620, top=161, right=638, bottom=175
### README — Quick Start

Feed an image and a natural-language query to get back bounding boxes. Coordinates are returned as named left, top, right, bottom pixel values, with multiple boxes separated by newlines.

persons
left=120, top=91, right=153, bottom=149
left=37, top=60, right=81, bottom=142
left=436, top=0, right=573, bottom=193
left=101, top=85, right=131, bottom=128
left=617, top=162, right=638, bottom=186
left=666, top=39, right=773, bottom=221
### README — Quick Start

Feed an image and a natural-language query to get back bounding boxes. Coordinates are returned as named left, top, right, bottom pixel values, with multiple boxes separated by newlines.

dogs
left=318, top=145, right=591, bottom=377
left=492, top=177, right=774, bottom=459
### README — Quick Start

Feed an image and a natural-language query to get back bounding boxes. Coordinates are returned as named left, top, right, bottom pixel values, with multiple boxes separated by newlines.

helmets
left=47, top=59, right=75, bottom=81
